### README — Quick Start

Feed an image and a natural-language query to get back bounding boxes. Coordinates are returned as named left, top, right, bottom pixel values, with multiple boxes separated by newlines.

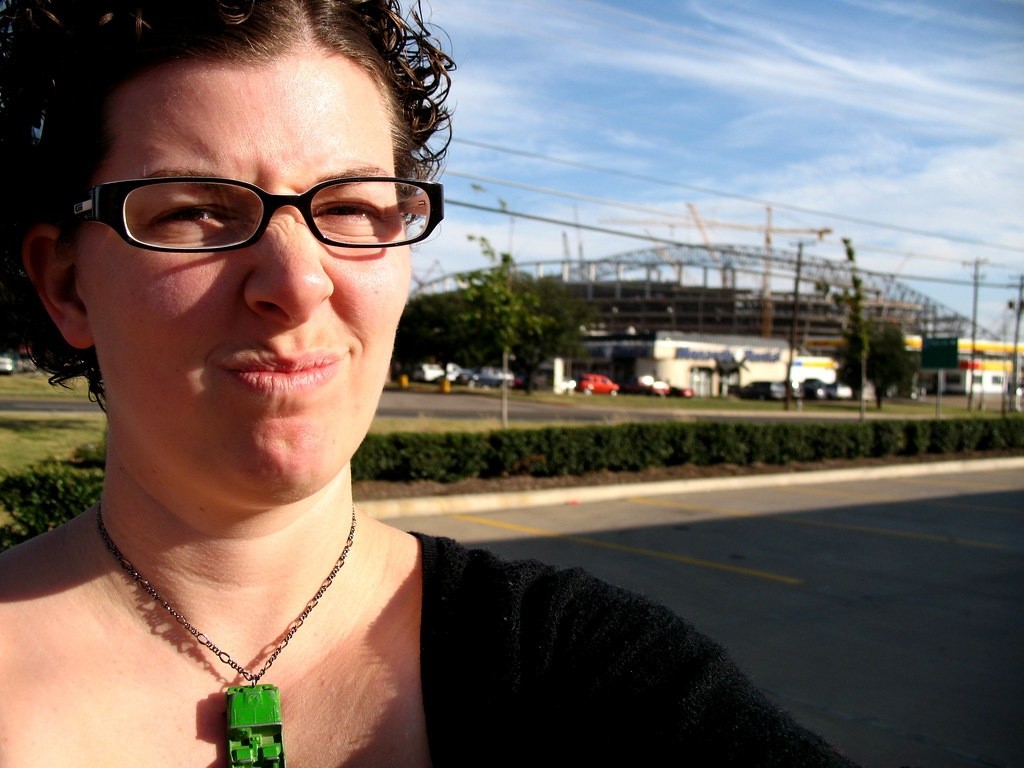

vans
left=574, top=372, right=620, bottom=397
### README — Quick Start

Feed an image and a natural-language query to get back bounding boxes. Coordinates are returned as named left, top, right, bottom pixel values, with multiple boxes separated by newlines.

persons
left=0, top=0, right=859, bottom=768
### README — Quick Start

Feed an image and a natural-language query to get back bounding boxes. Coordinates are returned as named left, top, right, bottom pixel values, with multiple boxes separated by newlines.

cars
left=12, top=354, right=38, bottom=373
left=620, top=377, right=693, bottom=398
left=0, top=353, right=14, bottom=373
left=413, top=360, right=525, bottom=390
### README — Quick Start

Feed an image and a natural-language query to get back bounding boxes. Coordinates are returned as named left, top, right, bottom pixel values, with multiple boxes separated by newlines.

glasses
left=59, top=174, right=446, bottom=253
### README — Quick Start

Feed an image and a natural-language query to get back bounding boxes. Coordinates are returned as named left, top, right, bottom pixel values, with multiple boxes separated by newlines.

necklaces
left=95, top=500, right=357, bottom=768
